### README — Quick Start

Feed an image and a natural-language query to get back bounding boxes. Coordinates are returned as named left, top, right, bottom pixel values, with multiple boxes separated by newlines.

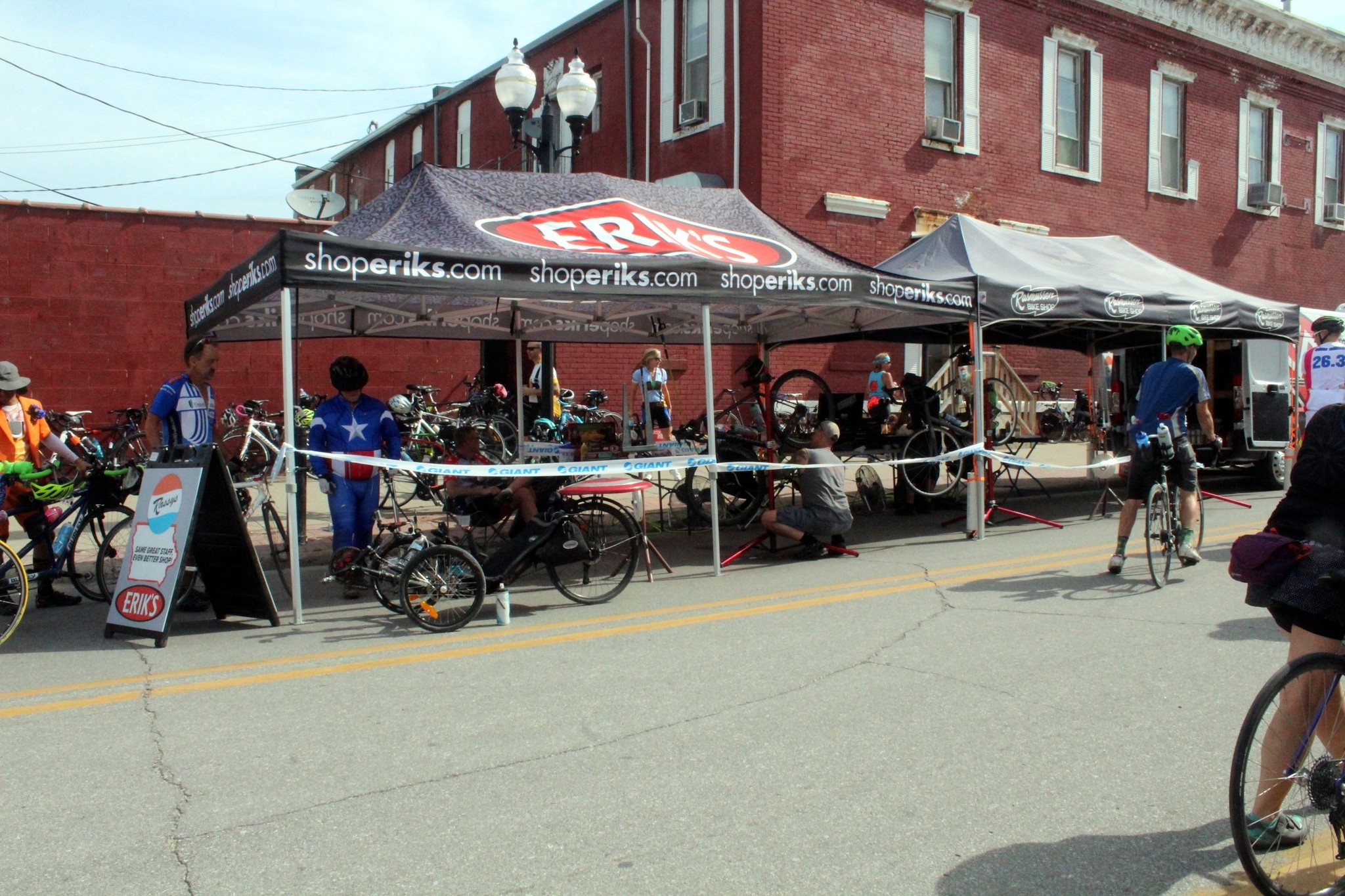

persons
left=522, top=342, right=562, bottom=427
left=627, top=347, right=684, bottom=483
left=761, top=420, right=856, bottom=560
left=148, top=333, right=250, bottom=614
left=1106, top=324, right=1223, bottom=574
left=1297, top=314, right=1344, bottom=436
left=308, top=357, right=404, bottom=601
left=0, top=360, right=102, bottom=618
left=1242, top=403, right=1345, bottom=854
left=442, top=424, right=542, bottom=530
left=865, top=353, right=903, bottom=464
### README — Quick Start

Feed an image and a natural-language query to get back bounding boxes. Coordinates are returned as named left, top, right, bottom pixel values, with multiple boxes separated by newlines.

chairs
left=439, top=473, right=513, bottom=549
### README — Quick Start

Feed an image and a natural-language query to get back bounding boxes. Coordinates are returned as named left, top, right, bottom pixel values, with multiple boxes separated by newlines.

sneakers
left=1246, top=812, right=1311, bottom=850
left=1107, top=553, right=1127, bottom=574
left=1178, top=542, right=1203, bottom=563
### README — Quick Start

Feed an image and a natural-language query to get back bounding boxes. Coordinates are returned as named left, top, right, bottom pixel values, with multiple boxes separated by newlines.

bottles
left=496, top=583, right=509, bottom=625
left=1135, top=430, right=1152, bottom=448
left=52, top=522, right=74, bottom=558
left=1157, top=422, right=1175, bottom=459
left=580, top=442, right=587, bottom=461
left=44, top=506, right=62, bottom=525
left=92, top=437, right=103, bottom=458
left=397, top=535, right=426, bottom=567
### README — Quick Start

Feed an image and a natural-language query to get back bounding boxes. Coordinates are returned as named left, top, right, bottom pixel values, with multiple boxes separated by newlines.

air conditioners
left=1324, top=203, right=1345, bottom=222
left=678, top=99, right=702, bottom=124
left=929, top=116, right=961, bottom=142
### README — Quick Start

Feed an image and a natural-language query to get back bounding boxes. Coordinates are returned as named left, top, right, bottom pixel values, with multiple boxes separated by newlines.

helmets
left=1042, top=380, right=1056, bottom=390
left=534, top=418, right=557, bottom=442
left=220, top=408, right=237, bottom=428
left=1166, top=324, right=1203, bottom=346
left=294, top=408, right=315, bottom=428
left=389, top=394, right=411, bottom=414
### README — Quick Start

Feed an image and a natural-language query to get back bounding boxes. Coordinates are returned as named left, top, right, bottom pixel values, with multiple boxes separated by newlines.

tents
left=178, top=157, right=986, bottom=639
left=844, top=209, right=1302, bottom=537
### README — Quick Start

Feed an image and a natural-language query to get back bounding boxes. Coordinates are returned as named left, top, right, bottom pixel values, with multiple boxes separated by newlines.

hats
left=1311, top=316, right=1345, bottom=332
left=0, top=360, right=31, bottom=390
left=818, top=420, right=840, bottom=444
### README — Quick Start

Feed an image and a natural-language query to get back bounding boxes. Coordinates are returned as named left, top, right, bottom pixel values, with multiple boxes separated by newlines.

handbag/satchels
left=1227, top=532, right=1308, bottom=584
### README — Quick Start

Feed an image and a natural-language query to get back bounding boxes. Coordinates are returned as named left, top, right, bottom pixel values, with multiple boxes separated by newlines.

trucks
left=1104, top=306, right=1345, bottom=489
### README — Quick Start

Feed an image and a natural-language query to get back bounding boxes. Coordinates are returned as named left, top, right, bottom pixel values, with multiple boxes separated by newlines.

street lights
left=493, top=35, right=598, bottom=427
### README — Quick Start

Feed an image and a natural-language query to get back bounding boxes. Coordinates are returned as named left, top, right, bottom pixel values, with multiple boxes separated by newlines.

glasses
left=526, top=347, right=540, bottom=351
left=1311, top=329, right=1327, bottom=338
left=186, top=338, right=219, bottom=357
left=468, top=435, right=482, bottom=441
left=881, top=360, right=891, bottom=364
left=649, top=357, right=662, bottom=361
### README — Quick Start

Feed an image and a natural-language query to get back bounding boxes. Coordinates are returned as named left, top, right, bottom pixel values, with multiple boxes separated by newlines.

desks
left=833, top=435, right=943, bottom=510
left=987, top=434, right=1053, bottom=504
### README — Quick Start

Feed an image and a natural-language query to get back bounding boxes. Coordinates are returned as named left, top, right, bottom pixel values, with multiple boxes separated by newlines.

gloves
left=1210, top=435, right=1223, bottom=452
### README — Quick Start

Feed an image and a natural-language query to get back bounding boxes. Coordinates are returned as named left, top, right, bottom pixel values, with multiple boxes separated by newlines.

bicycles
left=1, top=377, right=1106, bottom=650
left=1142, top=432, right=1222, bottom=588
left=1229, top=643, right=1345, bottom=894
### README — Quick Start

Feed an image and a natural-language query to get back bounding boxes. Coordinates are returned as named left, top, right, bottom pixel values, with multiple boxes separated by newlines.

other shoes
left=490, top=489, right=513, bottom=507
left=916, top=500, right=931, bottom=514
left=830, top=535, right=846, bottom=557
left=793, top=540, right=829, bottom=561
left=36, top=591, right=82, bottom=608
left=0, top=588, right=19, bottom=616
left=894, top=503, right=914, bottom=515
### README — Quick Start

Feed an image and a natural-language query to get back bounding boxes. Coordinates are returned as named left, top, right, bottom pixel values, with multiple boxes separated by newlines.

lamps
left=1248, top=182, right=1283, bottom=206
